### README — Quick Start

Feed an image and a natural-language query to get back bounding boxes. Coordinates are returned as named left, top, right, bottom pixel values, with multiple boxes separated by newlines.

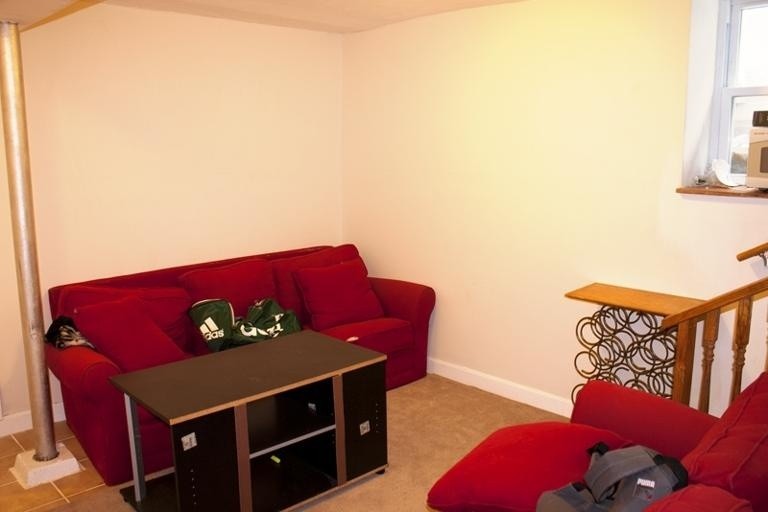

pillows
left=640, top=482, right=753, bottom=512
left=680, top=371, right=767, bottom=512
left=267, top=244, right=369, bottom=315
left=58, top=284, right=191, bottom=337
left=426, top=422, right=633, bottom=512
left=292, top=259, right=384, bottom=326
left=66, top=294, right=189, bottom=375
left=177, top=257, right=281, bottom=322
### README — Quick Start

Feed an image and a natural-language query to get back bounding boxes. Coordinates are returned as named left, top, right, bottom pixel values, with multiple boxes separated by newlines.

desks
left=564, top=282, right=708, bottom=407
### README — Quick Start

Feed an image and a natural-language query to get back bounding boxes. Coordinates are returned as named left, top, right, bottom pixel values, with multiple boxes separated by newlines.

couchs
left=45, top=244, right=436, bottom=487
left=569, top=372, right=767, bottom=512
